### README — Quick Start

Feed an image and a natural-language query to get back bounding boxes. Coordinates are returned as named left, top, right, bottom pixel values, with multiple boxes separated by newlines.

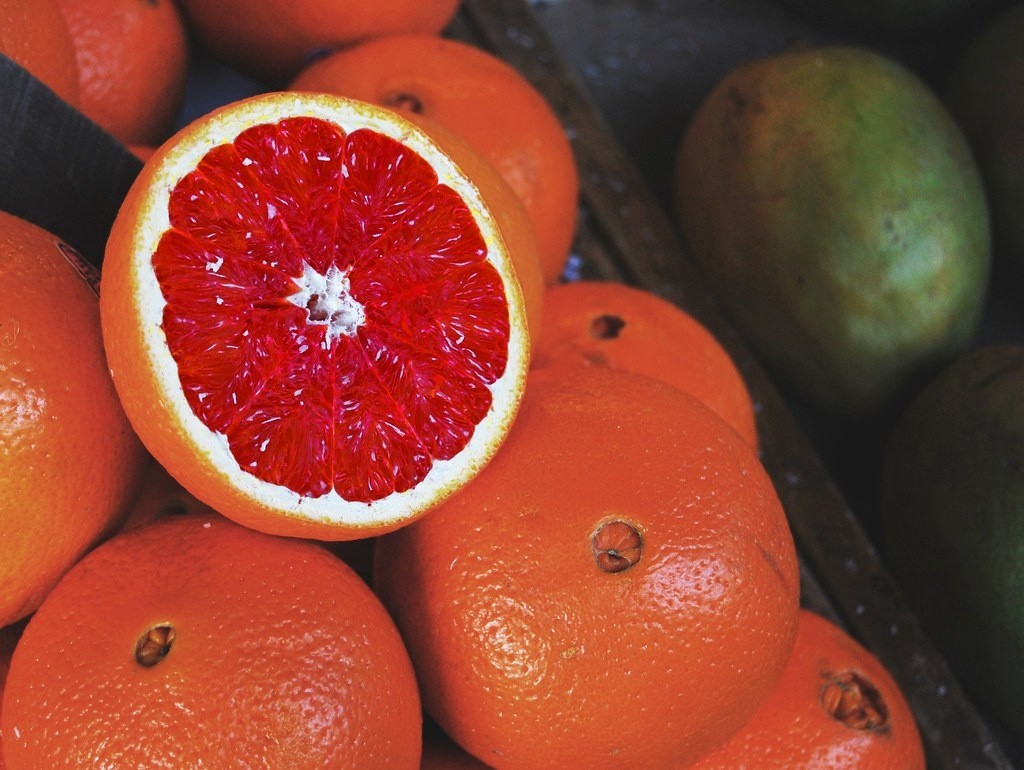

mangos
left=878, top=324, right=1024, bottom=751
left=669, top=40, right=990, bottom=417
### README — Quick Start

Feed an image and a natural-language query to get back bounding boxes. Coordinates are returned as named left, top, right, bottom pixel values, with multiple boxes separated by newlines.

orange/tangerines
left=0, top=0, right=923, bottom=770
